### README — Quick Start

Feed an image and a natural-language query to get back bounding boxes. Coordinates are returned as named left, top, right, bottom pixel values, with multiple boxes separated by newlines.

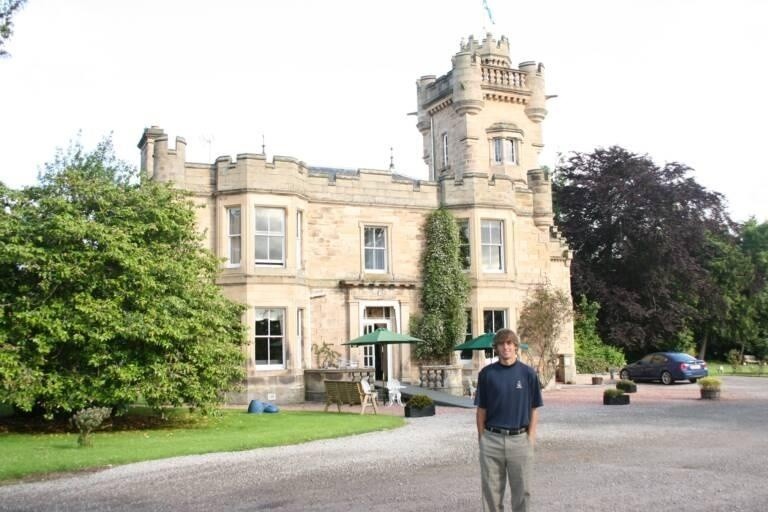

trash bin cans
left=558, top=355, right=576, bottom=383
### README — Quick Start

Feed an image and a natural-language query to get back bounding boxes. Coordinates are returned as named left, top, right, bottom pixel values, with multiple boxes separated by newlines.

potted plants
left=405, top=394, right=435, bottom=418
left=696, top=379, right=721, bottom=399
left=603, top=379, right=637, bottom=404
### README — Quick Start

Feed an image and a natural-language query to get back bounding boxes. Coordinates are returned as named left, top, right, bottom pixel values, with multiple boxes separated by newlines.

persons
left=474, top=328, right=544, bottom=511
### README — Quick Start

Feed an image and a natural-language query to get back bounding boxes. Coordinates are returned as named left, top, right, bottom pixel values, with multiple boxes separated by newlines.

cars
left=619, top=351, right=708, bottom=385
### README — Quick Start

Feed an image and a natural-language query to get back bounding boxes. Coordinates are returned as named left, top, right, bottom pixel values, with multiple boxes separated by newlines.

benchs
left=324, top=380, right=379, bottom=417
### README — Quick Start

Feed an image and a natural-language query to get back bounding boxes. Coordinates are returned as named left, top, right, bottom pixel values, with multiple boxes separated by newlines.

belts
left=485, top=425, right=528, bottom=435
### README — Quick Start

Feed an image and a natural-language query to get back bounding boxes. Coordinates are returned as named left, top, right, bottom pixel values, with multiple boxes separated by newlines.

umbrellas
left=341, top=332, right=426, bottom=406
left=452, top=330, right=529, bottom=364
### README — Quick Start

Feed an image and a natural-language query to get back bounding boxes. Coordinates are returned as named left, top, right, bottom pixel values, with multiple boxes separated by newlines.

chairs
left=360, top=379, right=407, bottom=407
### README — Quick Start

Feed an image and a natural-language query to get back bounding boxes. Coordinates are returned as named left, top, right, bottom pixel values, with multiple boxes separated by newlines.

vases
left=592, top=377, right=603, bottom=385
